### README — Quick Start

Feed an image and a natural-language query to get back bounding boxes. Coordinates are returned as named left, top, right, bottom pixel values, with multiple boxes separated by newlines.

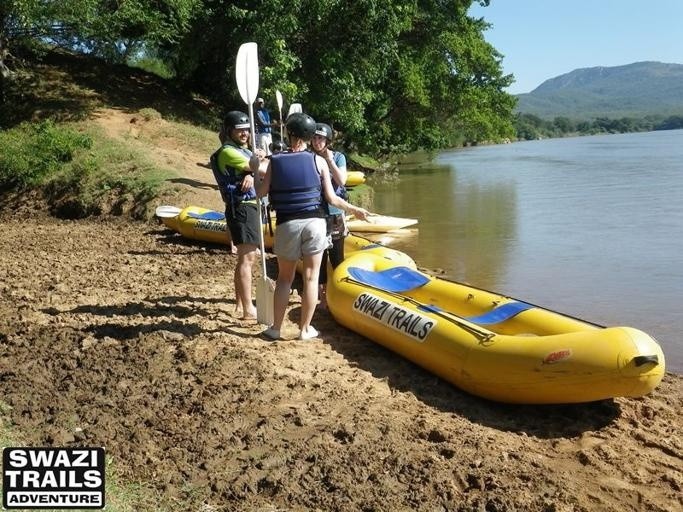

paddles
left=235, top=41, right=275, bottom=326
left=155, top=204, right=183, bottom=218
left=287, top=103, right=302, bottom=117
left=275, top=89, right=284, bottom=152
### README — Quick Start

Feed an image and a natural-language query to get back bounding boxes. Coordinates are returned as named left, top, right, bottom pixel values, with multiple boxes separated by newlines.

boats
left=195, top=160, right=367, bottom=190
left=156, top=202, right=420, bottom=258
left=323, top=244, right=665, bottom=405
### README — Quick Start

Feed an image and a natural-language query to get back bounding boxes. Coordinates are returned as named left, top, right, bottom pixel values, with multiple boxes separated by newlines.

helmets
left=222, top=111, right=250, bottom=135
left=285, top=113, right=315, bottom=142
left=313, top=122, right=332, bottom=144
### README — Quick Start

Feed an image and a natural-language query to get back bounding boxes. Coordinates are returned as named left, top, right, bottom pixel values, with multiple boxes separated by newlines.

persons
left=253, top=96, right=278, bottom=156
left=209, top=110, right=375, bottom=341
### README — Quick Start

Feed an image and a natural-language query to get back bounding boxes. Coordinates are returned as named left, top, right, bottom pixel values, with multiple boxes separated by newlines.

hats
left=256, top=97, right=264, bottom=104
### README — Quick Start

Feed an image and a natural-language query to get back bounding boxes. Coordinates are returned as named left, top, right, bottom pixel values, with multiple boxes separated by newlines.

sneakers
left=298, top=326, right=318, bottom=339
left=262, top=327, right=280, bottom=339
